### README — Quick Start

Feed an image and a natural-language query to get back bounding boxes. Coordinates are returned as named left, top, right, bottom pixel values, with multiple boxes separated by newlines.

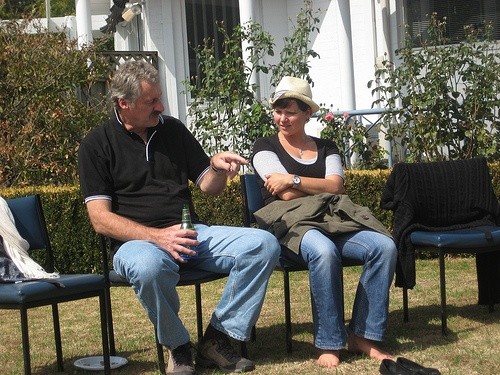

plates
left=74, top=356, right=129, bottom=370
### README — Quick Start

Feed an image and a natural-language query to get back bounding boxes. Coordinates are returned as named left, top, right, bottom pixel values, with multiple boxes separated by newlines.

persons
left=78, top=59, right=280, bottom=375
left=251, top=76, right=398, bottom=368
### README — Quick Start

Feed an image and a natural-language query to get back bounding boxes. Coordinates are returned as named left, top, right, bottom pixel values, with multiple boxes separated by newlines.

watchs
left=293, top=174, right=301, bottom=189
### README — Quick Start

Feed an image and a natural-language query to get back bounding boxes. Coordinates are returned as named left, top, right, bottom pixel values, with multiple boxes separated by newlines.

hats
left=269, top=76, right=320, bottom=114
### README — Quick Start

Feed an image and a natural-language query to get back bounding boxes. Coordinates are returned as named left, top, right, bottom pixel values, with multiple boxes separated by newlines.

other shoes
left=379, top=359, right=414, bottom=375
left=396, top=356, right=441, bottom=375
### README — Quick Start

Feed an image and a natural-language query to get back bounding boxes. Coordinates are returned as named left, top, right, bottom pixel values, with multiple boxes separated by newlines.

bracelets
left=212, top=166, right=222, bottom=172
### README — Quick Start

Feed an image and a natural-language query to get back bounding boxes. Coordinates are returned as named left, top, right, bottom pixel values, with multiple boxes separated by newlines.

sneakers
left=167, top=340, right=194, bottom=375
left=195, top=332, right=254, bottom=373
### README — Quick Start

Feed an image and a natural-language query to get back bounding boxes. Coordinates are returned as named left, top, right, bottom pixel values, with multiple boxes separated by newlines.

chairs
left=240, top=174, right=347, bottom=354
left=393, top=158, right=499, bottom=337
left=100, top=234, right=247, bottom=375
left=0, top=194, right=110, bottom=375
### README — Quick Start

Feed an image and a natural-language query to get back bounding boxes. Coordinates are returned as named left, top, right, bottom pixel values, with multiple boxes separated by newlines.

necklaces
left=288, top=134, right=307, bottom=155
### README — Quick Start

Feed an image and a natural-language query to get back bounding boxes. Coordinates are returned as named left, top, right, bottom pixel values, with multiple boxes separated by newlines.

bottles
left=178, top=203, right=199, bottom=259
